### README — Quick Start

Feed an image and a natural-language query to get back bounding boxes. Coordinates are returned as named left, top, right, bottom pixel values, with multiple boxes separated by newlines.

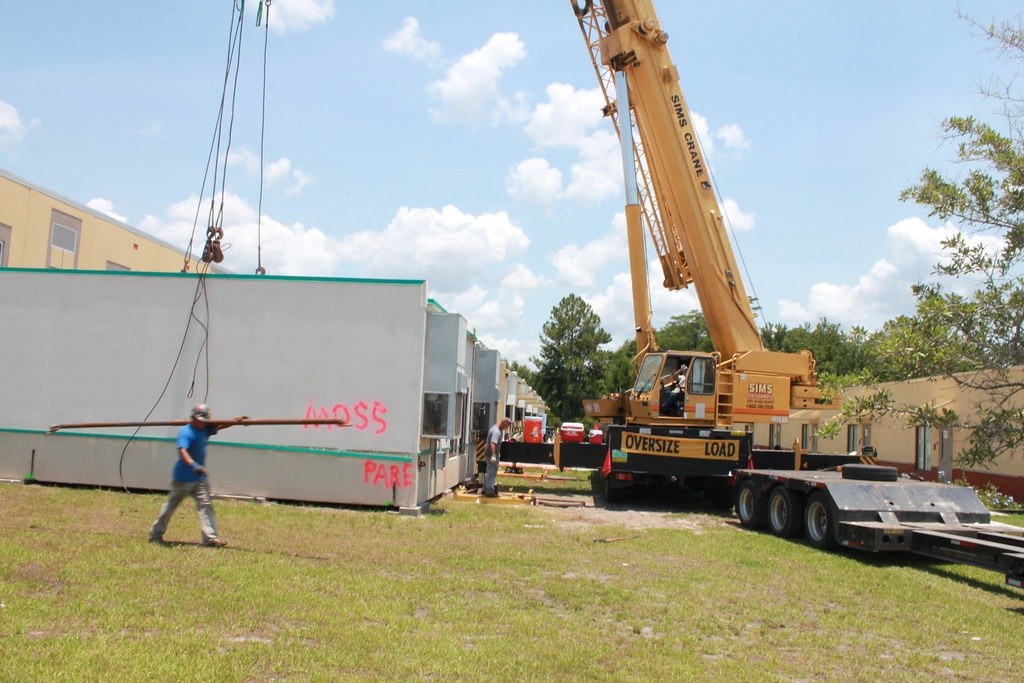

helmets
left=193, top=403, right=213, bottom=422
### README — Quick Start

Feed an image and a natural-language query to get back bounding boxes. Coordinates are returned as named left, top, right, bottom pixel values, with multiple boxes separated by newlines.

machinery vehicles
left=571, top=1, right=842, bottom=512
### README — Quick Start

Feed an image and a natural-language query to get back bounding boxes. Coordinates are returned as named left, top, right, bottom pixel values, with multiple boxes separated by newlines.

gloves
left=490, top=455, right=496, bottom=464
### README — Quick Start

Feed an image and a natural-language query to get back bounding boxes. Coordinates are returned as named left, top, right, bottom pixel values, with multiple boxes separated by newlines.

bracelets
left=193, top=462, right=198, bottom=472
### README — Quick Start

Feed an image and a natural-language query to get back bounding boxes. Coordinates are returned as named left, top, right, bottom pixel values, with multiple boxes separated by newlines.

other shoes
left=206, top=538, right=227, bottom=548
left=149, top=536, right=164, bottom=543
left=485, top=492, right=498, bottom=498
left=481, top=490, right=486, bottom=494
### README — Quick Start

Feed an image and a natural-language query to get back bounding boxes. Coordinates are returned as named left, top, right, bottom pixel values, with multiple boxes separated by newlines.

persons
left=149, top=404, right=250, bottom=547
left=662, top=364, right=688, bottom=410
left=481, top=417, right=511, bottom=497
